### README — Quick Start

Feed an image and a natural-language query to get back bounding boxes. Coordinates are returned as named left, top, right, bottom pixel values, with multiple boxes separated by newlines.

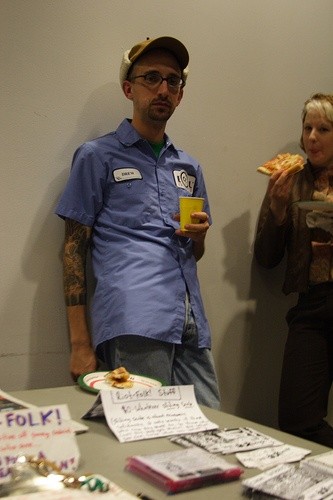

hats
left=119, top=36, right=189, bottom=89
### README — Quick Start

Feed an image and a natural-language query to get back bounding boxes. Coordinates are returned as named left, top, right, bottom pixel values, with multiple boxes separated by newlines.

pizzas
left=257, top=153, right=304, bottom=177
left=105, top=367, right=133, bottom=388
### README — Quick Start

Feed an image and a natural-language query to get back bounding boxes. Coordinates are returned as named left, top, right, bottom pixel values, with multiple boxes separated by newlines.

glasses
left=128, top=71, right=182, bottom=87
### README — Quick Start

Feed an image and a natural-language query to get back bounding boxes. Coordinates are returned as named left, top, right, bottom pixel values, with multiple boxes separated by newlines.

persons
left=253, top=94, right=333, bottom=449
left=55, top=36, right=222, bottom=411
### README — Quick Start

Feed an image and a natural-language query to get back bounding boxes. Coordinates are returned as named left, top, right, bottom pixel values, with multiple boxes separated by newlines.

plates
left=78, top=371, right=169, bottom=395
left=292, top=200, right=332, bottom=212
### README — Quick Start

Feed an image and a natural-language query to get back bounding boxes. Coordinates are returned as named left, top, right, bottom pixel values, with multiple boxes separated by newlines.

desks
left=0, top=386, right=333, bottom=500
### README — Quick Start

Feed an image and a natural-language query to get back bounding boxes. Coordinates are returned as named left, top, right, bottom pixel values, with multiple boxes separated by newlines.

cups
left=178, top=196, right=205, bottom=232
